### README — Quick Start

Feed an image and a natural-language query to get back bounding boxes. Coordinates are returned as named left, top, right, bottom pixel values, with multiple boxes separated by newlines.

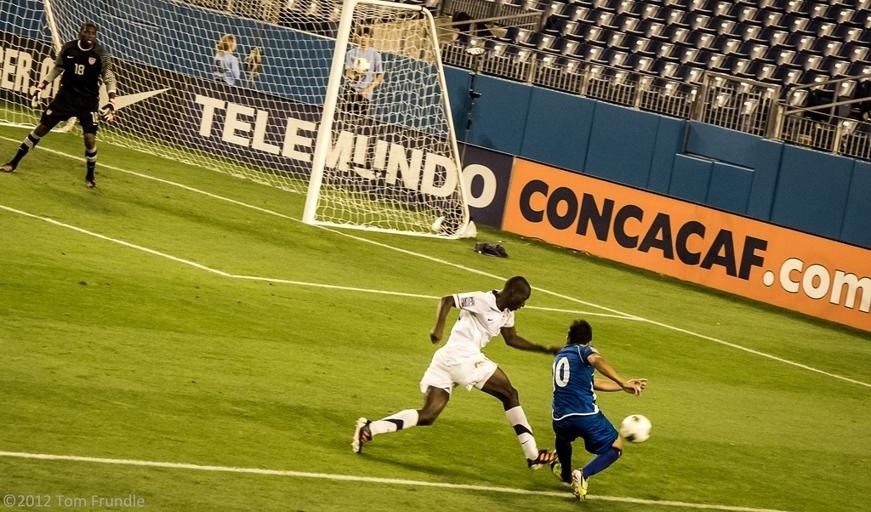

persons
left=339, top=26, right=382, bottom=114
left=548, top=319, right=647, bottom=501
left=0, top=22, right=116, bottom=188
left=351, top=274, right=559, bottom=470
left=214, top=33, right=241, bottom=84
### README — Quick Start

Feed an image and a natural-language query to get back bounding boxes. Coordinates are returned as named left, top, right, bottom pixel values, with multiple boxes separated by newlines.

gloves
left=100, top=99, right=115, bottom=122
left=31, top=83, right=47, bottom=107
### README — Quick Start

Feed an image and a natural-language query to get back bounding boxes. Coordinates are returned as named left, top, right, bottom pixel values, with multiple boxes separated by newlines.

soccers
left=619, top=414, right=652, bottom=443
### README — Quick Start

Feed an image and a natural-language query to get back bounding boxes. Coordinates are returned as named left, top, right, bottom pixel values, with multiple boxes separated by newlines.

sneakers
left=526, top=447, right=562, bottom=472
left=551, top=461, right=574, bottom=488
left=0, top=161, right=17, bottom=174
left=571, top=467, right=590, bottom=503
left=84, top=174, right=97, bottom=190
left=349, top=415, right=373, bottom=456
